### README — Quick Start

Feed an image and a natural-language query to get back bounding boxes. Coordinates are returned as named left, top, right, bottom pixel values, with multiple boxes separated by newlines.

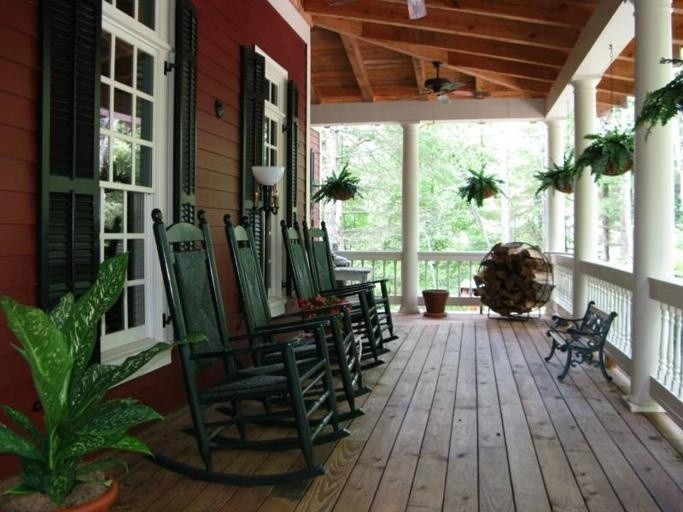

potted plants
left=309, top=161, right=364, bottom=205
left=0, top=251, right=210, bottom=512
left=457, top=161, right=509, bottom=208
left=532, top=125, right=635, bottom=198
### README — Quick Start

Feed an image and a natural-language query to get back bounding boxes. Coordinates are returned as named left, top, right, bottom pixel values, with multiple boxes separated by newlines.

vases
left=422, top=289, right=448, bottom=318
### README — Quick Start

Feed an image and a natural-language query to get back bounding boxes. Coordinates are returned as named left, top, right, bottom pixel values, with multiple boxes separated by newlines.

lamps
left=423, top=62, right=452, bottom=103
left=250, top=165, right=285, bottom=211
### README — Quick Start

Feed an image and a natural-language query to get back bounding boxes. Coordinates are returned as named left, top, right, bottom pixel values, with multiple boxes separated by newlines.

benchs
left=545, top=300, right=618, bottom=382
